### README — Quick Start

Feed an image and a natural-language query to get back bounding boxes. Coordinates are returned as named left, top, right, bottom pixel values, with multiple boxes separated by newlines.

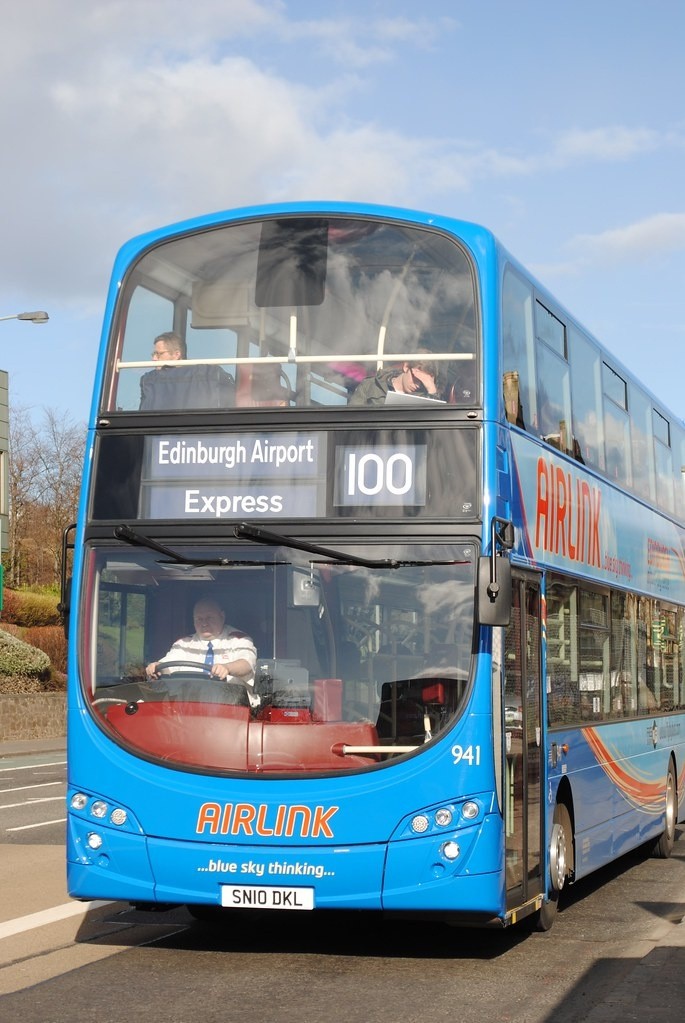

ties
left=203, top=641, right=214, bottom=674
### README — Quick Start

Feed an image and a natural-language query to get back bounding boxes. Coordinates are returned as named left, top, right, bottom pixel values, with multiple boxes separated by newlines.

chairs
left=337, top=642, right=461, bottom=715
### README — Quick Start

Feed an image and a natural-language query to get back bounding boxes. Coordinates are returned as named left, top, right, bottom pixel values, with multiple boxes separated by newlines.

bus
left=55, top=200, right=684, bottom=933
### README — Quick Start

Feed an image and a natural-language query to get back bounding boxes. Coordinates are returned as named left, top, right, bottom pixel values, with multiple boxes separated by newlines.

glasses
left=151, top=350, right=173, bottom=358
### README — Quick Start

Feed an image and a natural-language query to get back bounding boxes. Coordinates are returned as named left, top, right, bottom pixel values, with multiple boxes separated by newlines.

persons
left=147, top=599, right=261, bottom=710
left=347, top=349, right=443, bottom=408
left=137, top=332, right=205, bottom=411
